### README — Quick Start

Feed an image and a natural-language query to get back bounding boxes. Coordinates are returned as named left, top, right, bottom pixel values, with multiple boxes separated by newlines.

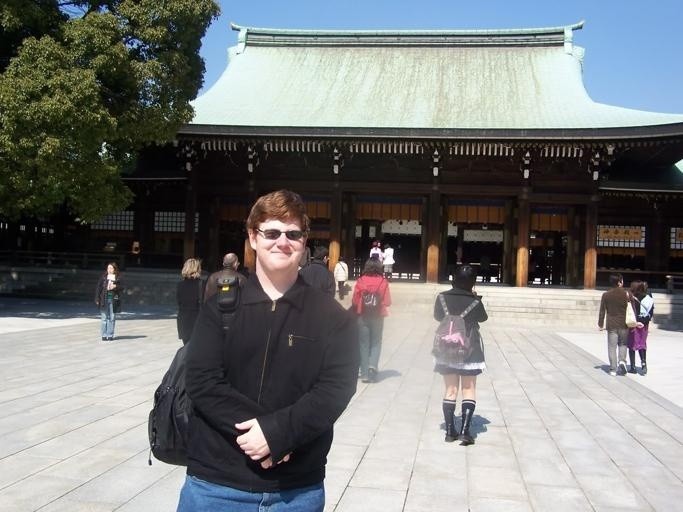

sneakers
left=109, top=337, right=113, bottom=342
left=366, top=368, right=378, bottom=382
left=619, top=361, right=628, bottom=374
left=609, top=370, right=618, bottom=376
left=101, top=337, right=108, bottom=341
left=629, top=366, right=636, bottom=373
left=642, top=363, right=648, bottom=370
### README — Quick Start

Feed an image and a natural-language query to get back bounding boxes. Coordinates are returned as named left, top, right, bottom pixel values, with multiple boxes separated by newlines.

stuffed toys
left=441, top=321, right=465, bottom=346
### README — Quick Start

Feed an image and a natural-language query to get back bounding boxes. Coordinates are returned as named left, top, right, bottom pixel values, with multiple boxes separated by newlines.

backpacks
left=432, top=292, right=481, bottom=360
left=145, top=277, right=240, bottom=467
left=359, top=278, right=385, bottom=316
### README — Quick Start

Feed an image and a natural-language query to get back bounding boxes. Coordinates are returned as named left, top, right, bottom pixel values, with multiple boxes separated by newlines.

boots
left=459, top=408, right=474, bottom=444
left=444, top=407, right=459, bottom=441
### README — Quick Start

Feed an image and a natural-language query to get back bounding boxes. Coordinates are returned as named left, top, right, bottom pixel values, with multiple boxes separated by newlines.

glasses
left=255, top=228, right=306, bottom=240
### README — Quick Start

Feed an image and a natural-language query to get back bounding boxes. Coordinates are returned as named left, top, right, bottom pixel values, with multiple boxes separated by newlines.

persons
left=627, top=280, right=654, bottom=374
left=177, top=258, right=205, bottom=347
left=480, top=254, right=490, bottom=282
left=528, top=255, right=536, bottom=286
left=598, top=273, right=633, bottom=376
left=382, top=244, right=395, bottom=281
left=430, top=265, right=489, bottom=444
left=539, top=259, right=546, bottom=285
left=643, top=282, right=653, bottom=298
left=298, top=246, right=335, bottom=299
left=352, top=258, right=392, bottom=382
left=334, top=256, right=349, bottom=301
left=204, top=253, right=247, bottom=302
left=94, top=262, right=121, bottom=341
left=369, top=240, right=384, bottom=262
left=406, top=272, right=414, bottom=280
left=488, top=265, right=493, bottom=283
left=174, top=190, right=359, bottom=512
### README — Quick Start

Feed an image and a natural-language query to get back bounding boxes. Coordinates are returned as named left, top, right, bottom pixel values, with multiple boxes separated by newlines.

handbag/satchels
left=623, top=290, right=637, bottom=329
